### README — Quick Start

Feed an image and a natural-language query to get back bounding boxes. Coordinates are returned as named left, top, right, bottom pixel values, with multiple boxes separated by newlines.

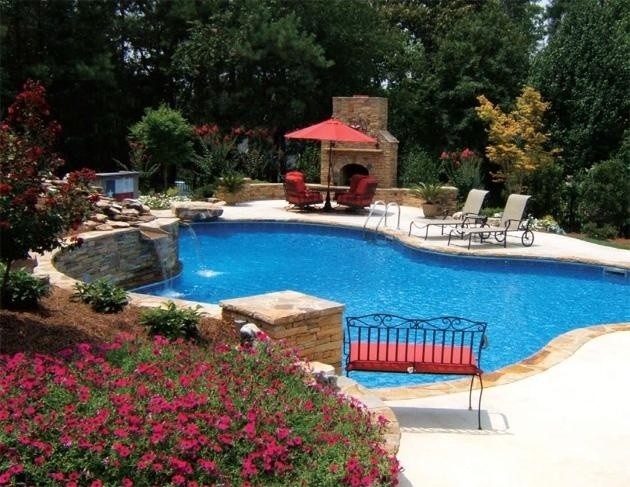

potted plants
left=414, top=177, right=445, bottom=219
left=214, top=170, right=247, bottom=206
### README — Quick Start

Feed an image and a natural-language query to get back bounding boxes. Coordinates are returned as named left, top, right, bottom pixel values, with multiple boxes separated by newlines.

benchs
left=346, top=313, right=487, bottom=429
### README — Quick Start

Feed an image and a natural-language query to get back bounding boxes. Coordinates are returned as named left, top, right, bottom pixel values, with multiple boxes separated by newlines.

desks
left=304, top=181, right=350, bottom=212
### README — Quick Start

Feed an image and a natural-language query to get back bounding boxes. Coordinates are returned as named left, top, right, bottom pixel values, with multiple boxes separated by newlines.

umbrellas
left=284, top=116, right=377, bottom=212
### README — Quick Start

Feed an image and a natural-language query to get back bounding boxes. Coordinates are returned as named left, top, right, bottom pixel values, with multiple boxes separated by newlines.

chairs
left=281, top=171, right=323, bottom=211
left=449, top=192, right=533, bottom=250
left=336, top=174, right=376, bottom=212
left=408, top=189, right=491, bottom=240
